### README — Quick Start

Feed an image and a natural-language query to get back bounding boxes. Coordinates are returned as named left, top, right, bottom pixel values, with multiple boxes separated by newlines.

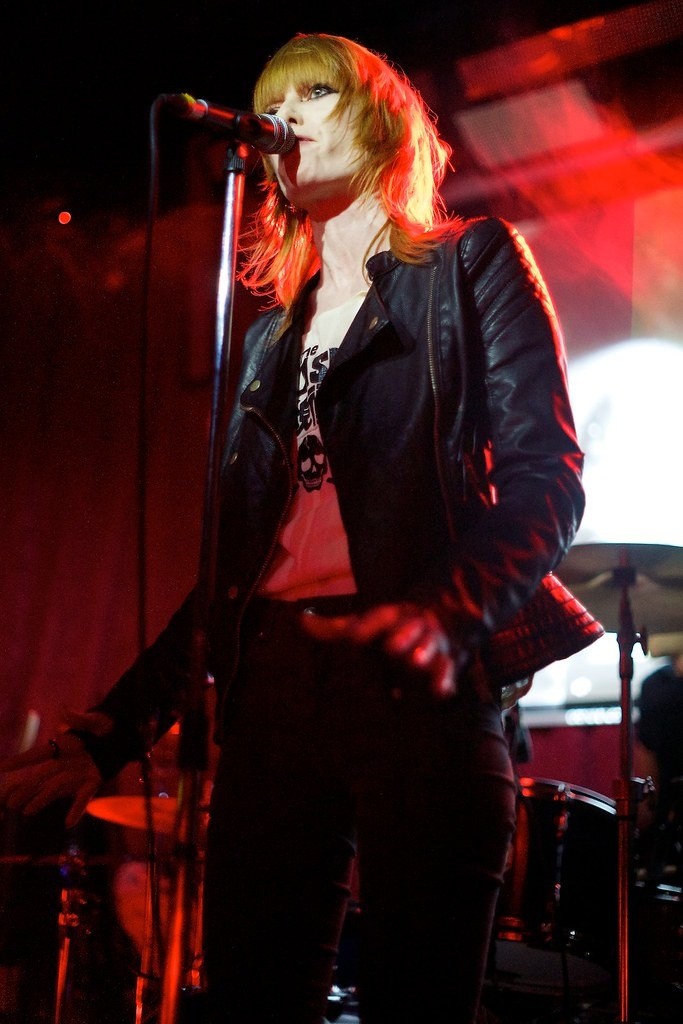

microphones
left=164, top=92, right=295, bottom=154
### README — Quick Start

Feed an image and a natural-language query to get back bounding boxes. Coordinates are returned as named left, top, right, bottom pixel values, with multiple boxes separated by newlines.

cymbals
left=83, top=792, right=210, bottom=841
left=551, top=543, right=683, bottom=636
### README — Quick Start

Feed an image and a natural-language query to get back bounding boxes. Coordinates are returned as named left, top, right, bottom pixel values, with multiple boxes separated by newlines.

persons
left=0, top=34, right=585, bottom=1024
left=637, top=635, right=683, bottom=867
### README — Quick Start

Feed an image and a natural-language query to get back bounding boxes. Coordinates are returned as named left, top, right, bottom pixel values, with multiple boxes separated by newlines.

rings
left=49, top=740, right=60, bottom=761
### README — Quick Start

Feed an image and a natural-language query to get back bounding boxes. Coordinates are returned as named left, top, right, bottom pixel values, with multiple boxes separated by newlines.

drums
left=484, top=774, right=639, bottom=997
